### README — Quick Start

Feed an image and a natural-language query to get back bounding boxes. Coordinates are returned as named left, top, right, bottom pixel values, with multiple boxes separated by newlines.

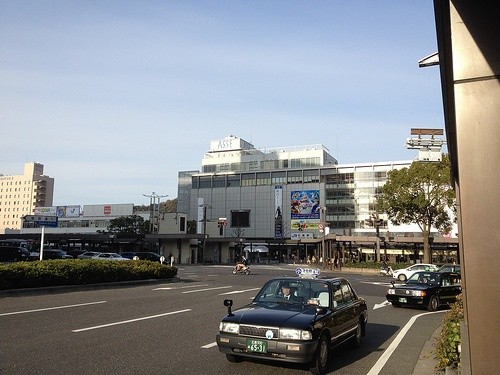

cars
left=386, top=271, right=462, bottom=310
left=0, top=239, right=164, bottom=263
left=393, top=264, right=439, bottom=279
left=437, top=265, right=461, bottom=274
left=215, top=268, right=368, bottom=375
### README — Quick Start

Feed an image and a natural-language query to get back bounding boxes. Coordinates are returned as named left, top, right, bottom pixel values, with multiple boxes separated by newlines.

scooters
left=381, top=265, right=393, bottom=277
left=233, top=263, right=250, bottom=275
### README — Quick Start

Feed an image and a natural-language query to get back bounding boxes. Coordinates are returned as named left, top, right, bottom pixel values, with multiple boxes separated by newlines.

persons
left=311, top=255, right=339, bottom=268
left=380, top=260, right=390, bottom=274
left=236, top=255, right=248, bottom=271
left=279, top=280, right=298, bottom=304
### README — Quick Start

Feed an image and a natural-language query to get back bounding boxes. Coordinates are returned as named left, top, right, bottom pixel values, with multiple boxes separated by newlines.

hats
left=282, top=283, right=289, bottom=287
